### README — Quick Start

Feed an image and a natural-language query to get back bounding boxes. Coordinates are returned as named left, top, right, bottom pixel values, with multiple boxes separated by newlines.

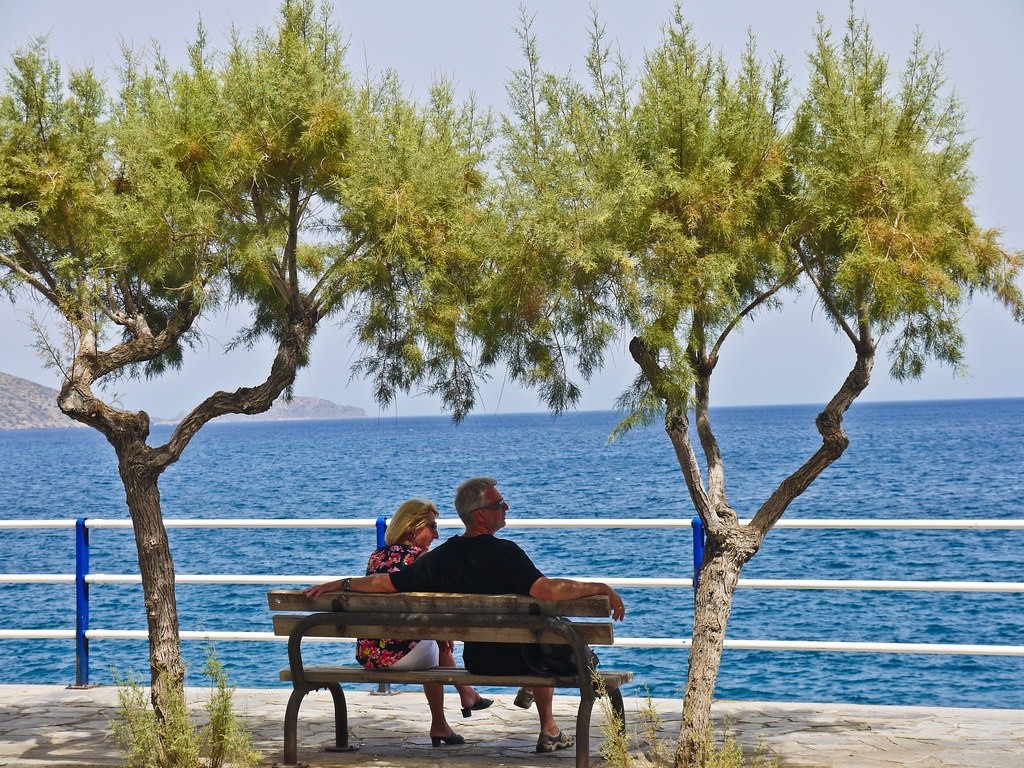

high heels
left=461, top=697, right=493, bottom=717
left=431, top=731, right=465, bottom=747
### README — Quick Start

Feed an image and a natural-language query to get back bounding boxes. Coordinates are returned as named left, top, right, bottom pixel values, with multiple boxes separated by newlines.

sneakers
left=536, top=731, right=575, bottom=752
left=514, top=688, right=536, bottom=709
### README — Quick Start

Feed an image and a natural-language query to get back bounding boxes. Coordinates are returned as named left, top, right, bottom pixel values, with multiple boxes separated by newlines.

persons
left=302, top=478, right=625, bottom=755
left=356, top=499, right=494, bottom=746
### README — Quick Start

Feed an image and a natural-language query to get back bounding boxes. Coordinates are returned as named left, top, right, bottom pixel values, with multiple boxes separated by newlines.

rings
left=618, top=606, right=622, bottom=610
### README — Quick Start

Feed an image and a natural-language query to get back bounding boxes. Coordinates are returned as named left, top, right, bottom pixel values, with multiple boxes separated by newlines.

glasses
left=469, top=497, right=504, bottom=514
left=425, top=522, right=437, bottom=531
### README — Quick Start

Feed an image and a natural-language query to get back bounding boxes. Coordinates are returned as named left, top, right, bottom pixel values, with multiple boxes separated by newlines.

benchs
left=267, top=590, right=634, bottom=768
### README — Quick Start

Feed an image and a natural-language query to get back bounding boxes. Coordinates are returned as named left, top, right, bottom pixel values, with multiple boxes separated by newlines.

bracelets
left=342, top=578, right=351, bottom=592
